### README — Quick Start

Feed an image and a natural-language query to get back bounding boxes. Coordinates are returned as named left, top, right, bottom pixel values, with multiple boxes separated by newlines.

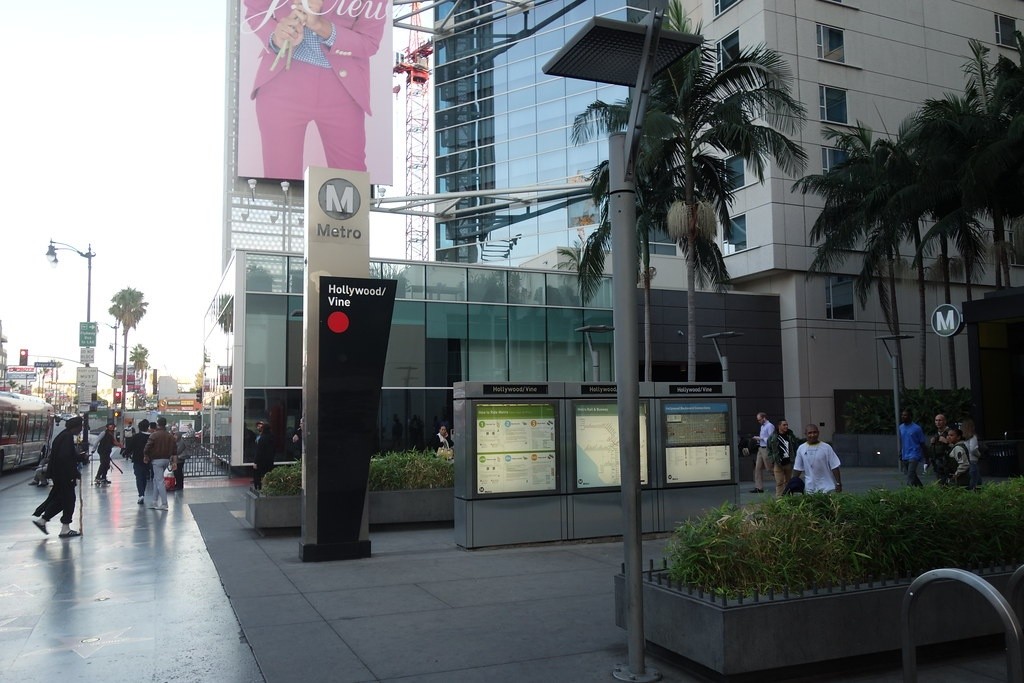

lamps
left=280, top=181, right=290, bottom=205
left=377, top=187, right=386, bottom=208
left=247, top=179, right=257, bottom=201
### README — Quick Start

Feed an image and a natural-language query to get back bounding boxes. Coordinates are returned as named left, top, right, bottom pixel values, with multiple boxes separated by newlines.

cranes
left=392, top=1, right=430, bottom=265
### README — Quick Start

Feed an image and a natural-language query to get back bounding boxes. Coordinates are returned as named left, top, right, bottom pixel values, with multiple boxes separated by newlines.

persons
left=392, top=414, right=448, bottom=450
left=766, top=420, right=799, bottom=499
left=793, top=424, right=842, bottom=495
left=243, top=0, right=389, bottom=181
left=750, top=412, right=775, bottom=493
left=252, top=422, right=275, bottom=489
left=433, top=426, right=454, bottom=452
left=187, top=423, right=195, bottom=458
left=91, top=423, right=123, bottom=484
left=33, top=417, right=88, bottom=536
left=292, top=419, right=302, bottom=461
left=930, top=414, right=983, bottom=492
left=123, top=417, right=187, bottom=510
left=28, top=454, right=50, bottom=487
left=899, top=410, right=929, bottom=488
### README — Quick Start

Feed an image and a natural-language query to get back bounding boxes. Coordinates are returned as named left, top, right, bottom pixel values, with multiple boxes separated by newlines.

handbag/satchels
left=978, top=442, right=990, bottom=460
left=177, top=447, right=194, bottom=461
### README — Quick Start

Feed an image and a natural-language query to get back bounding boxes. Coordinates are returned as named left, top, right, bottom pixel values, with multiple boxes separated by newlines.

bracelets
left=837, top=482, right=841, bottom=485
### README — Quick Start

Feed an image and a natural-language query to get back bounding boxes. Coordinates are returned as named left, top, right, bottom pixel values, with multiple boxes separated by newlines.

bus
left=0, top=391, right=54, bottom=476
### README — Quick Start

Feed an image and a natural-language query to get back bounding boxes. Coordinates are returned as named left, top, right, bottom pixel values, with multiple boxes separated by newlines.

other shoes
left=36, top=483, right=48, bottom=487
left=148, top=502, right=158, bottom=509
left=137, top=495, right=146, bottom=505
left=102, top=479, right=111, bottom=484
left=155, top=504, right=169, bottom=512
left=28, top=481, right=39, bottom=486
left=93, top=478, right=101, bottom=483
left=749, top=488, right=763, bottom=494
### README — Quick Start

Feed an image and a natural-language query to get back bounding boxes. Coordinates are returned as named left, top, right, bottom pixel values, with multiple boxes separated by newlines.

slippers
left=59, top=529, right=81, bottom=538
left=32, top=520, right=49, bottom=535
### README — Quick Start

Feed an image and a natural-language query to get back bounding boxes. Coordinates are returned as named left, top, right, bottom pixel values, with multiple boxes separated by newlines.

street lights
left=704, top=332, right=745, bottom=382
left=46, top=239, right=96, bottom=465
left=875, top=335, right=915, bottom=472
left=575, top=325, right=615, bottom=383
left=543, top=7, right=703, bottom=683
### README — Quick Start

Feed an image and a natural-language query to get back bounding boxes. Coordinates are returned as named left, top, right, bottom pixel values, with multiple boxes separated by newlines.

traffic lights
left=19, top=349, right=28, bottom=366
left=113, top=392, right=121, bottom=404
left=113, top=412, right=120, bottom=417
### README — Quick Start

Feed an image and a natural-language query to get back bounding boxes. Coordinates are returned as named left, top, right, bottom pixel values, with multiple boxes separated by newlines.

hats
left=64, top=416, right=87, bottom=427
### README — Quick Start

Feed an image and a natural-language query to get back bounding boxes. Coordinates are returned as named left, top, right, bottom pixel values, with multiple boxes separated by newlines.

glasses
left=757, top=419, right=761, bottom=422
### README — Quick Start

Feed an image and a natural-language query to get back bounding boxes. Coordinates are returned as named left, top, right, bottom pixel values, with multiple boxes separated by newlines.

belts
left=758, top=445, right=768, bottom=449
